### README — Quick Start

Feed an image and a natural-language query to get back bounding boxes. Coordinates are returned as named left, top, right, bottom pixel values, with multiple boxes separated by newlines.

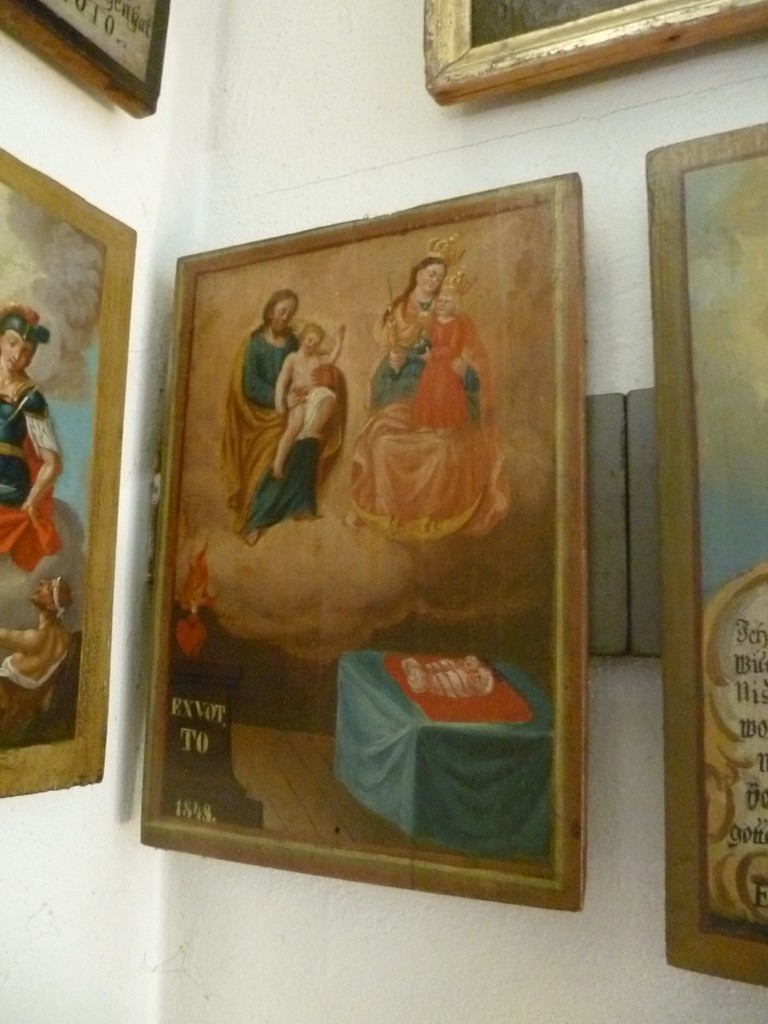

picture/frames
left=0, top=0, right=169, bottom=119
left=644, top=121, right=768, bottom=989
left=0, top=146, right=138, bottom=797
left=140, top=173, right=590, bottom=913
left=422, top=0, right=767, bottom=106
